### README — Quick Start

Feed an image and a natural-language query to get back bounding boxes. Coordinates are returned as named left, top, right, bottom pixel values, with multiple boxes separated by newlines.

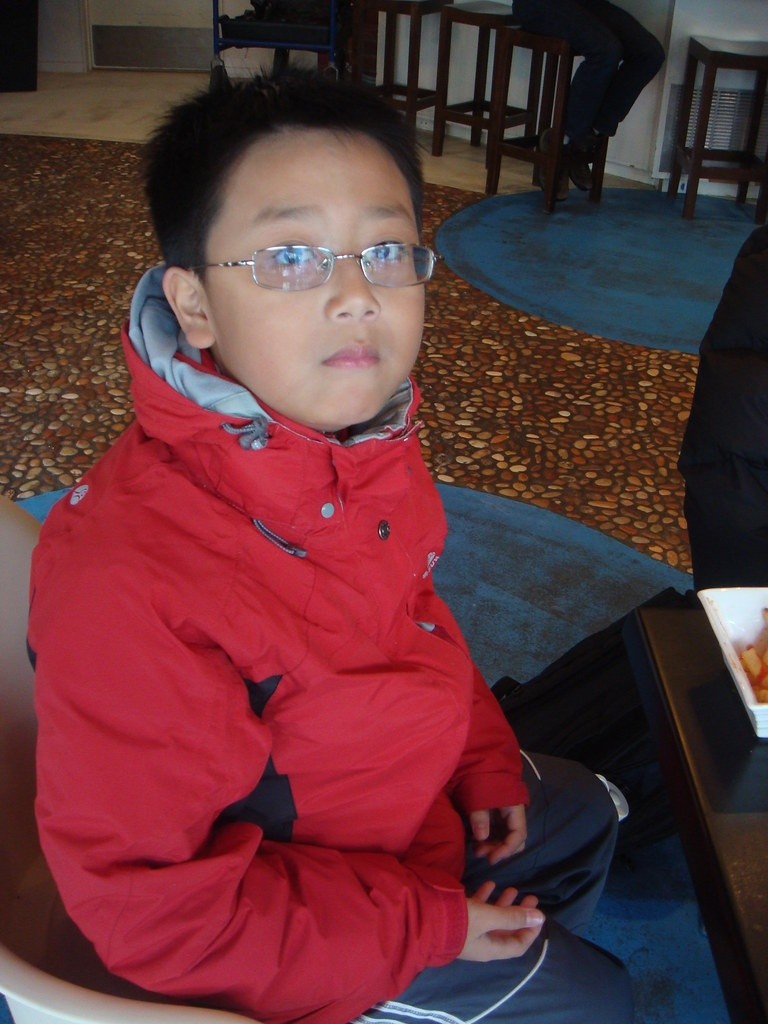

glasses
left=207, top=242, right=445, bottom=291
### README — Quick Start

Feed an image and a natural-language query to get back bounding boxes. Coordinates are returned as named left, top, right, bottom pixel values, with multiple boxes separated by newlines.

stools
left=353, top=0, right=609, bottom=215
left=668, top=36, right=768, bottom=219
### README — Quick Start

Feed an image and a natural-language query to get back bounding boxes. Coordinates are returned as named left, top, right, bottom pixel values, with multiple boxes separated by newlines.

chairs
left=213, top=0, right=342, bottom=91
left=0, top=481, right=694, bottom=1024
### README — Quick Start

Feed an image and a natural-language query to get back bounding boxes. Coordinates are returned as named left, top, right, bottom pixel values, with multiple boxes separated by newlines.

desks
left=640, top=606, right=768, bottom=1024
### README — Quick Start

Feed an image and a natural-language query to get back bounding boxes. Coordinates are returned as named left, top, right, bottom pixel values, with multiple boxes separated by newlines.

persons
left=513, top=0, right=665, bottom=191
left=26, top=62, right=633, bottom=1024
left=676, top=219, right=768, bottom=599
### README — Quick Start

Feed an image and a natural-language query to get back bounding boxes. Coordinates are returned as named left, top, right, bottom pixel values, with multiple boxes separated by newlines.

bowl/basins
left=697, top=587, right=768, bottom=737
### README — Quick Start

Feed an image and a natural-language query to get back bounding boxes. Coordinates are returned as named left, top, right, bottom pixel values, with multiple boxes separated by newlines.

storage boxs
left=697, top=587, right=768, bottom=737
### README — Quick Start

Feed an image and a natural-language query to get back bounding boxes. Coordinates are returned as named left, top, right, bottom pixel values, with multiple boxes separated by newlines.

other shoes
left=561, top=139, right=595, bottom=193
left=542, top=128, right=569, bottom=198
